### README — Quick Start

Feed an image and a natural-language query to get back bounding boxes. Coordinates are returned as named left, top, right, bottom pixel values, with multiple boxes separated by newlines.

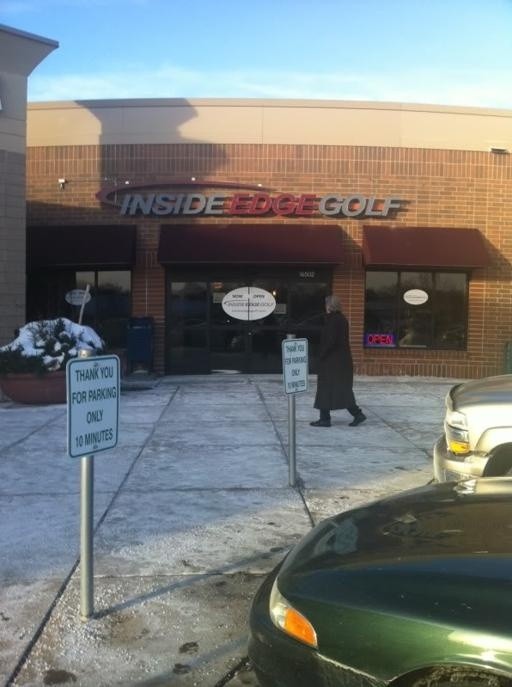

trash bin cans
left=127, top=317, right=154, bottom=368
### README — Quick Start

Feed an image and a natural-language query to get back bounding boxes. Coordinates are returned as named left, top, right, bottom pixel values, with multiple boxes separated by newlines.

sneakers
left=349, top=414, right=366, bottom=426
left=310, top=418, right=331, bottom=427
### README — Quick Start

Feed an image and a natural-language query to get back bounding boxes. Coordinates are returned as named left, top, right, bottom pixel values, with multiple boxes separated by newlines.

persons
left=263, top=315, right=281, bottom=357
left=308, top=295, right=367, bottom=427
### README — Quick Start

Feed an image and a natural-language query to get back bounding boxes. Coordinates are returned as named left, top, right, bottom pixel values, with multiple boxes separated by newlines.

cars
left=248, top=477, right=512, bottom=687
left=434, top=374, right=512, bottom=481
